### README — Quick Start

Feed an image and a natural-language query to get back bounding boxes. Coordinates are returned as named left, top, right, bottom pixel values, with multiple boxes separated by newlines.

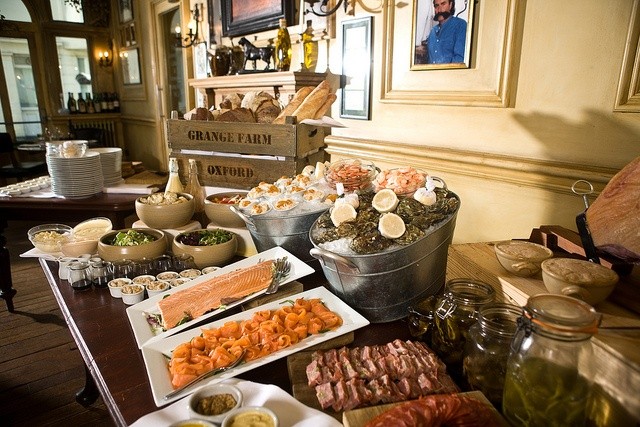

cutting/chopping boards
left=343, top=389, right=497, bottom=425
left=587, top=157, right=640, bottom=264
left=287, top=340, right=463, bottom=410
left=103, top=169, right=168, bottom=194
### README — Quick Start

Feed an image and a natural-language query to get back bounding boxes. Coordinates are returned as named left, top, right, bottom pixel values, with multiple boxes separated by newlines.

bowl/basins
left=57, top=240, right=98, bottom=256
left=156, top=271, right=178, bottom=281
left=45, top=140, right=87, bottom=158
left=108, top=278, right=130, bottom=298
left=72, top=217, right=112, bottom=240
left=170, top=278, right=191, bottom=287
left=165, top=418, right=214, bottom=427
left=325, top=158, right=375, bottom=188
left=132, top=275, right=155, bottom=285
left=145, top=281, right=170, bottom=297
left=376, top=166, right=429, bottom=196
left=135, top=191, right=196, bottom=228
left=186, top=382, right=244, bottom=422
left=172, top=229, right=238, bottom=269
left=120, top=284, right=145, bottom=305
left=221, top=405, right=279, bottom=427
left=98, top=227, right=168, bottom=262
left=541, top=256, right=618, bottom=307
left=27, top=224, right=72, bottom=252
left=492, top=239, right=551, bottom=278
left=204, top=191, right=251, bottom=227
left=180, top=269, right=202, bottom=279
left=203, top=266, right=221, bottom=274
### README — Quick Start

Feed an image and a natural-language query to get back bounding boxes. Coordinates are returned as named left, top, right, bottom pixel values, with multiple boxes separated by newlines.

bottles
left=275, top=19, right=293, bottom=70
left=230, top=46, right=246, bottom=74
left=184, top=159, right=205, bottom=212
left=206, top=44, right=229, bottom=76
left=57, top=92, right=120, bottom=113
left=503, top=293, right=602, bottom=426
left=432, top=277, right=496, bottom=357
left=165, top=158, right=184, bottom=193
left=302, top=20, right=318, bottom=72
left=463, top=303, right=523, bottom=410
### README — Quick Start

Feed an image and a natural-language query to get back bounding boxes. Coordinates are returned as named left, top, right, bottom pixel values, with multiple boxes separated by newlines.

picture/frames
left=409, top=0, right=480, bottom=71
left=220, top=0, right=294, bottom=37
left=379, top=0, right=526, bottom=109
left=299, top=0, right=336, bottom=41
left=612, top=0, right=640, bottom=112
left=339, top=16, right=372, bottom=121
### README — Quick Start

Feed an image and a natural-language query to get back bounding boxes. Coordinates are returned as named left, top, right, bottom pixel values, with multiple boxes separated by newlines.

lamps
left=187, top=23, right=192, bottom=42
left=119, top=52, right=123, bottom=68
left=104, top=51, right=109, bottom=65
left=98, top=51, right=103, bottom=65
left=124, top=53, right=128, bottom=70
left=175, top=26, right=181, bottom=48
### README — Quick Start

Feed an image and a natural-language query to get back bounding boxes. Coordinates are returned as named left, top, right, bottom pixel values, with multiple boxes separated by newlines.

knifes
left=571, top=181, right=600, bottom=266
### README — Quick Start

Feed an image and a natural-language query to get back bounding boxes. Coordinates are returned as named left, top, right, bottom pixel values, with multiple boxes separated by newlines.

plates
left=128, top=378, right=341, bottom=427
left=44, top=152, right=103, bottom=199
left=18, top=248, right=64, bottom=261
left=141, top=285, right=371, bottom=408
left=87, top=147, right=124, bottom=186
left=126, top=247, right=316, bottom=348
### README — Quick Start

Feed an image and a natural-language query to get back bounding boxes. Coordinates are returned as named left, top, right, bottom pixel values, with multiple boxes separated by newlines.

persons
left=419, top=0, right=468, bottom=64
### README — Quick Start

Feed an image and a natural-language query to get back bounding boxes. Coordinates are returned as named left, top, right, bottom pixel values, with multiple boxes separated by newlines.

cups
left=135, top=258, right=151, bottom=276
left=92, top=261, right=110, bottom=286
left=152, top=255, right=171, bottom=272
left=109, top=259, right=135, bottom=279
left=173, top=253, right=198, bottom=270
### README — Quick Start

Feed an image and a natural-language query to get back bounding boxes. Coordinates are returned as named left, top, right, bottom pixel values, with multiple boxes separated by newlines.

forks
left=265, top=258, right=291, bottom=294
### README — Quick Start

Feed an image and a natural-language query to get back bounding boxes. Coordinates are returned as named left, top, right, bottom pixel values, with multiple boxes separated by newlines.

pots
left=228, top=165, right=382, bottom=268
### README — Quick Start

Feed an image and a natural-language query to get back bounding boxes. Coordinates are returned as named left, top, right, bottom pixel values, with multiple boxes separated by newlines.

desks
left=0, top=171, right=169, bottom=313
left=35, top=254, right=466, bottom=427
left=188, top=70, right=327, bottom=125
left=48, top=112, right=128, bottom=162
left=448, top=240, right=640, bottom=374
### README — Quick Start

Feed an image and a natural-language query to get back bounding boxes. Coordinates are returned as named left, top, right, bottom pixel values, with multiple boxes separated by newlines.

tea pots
left=56, top=257, right=71, bottom=280
left=78, top=254, right=92, bottom=262
left=66, top=263, right=92, bottom=292
left=86, top=257, right=103, bottom=282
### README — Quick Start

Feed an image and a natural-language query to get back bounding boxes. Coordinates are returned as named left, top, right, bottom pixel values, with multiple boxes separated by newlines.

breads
left=271, top=79, right=339, bottom=125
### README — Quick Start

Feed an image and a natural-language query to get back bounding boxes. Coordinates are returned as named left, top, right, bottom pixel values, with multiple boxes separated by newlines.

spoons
left=164, top=350, right=251, bottom=399
